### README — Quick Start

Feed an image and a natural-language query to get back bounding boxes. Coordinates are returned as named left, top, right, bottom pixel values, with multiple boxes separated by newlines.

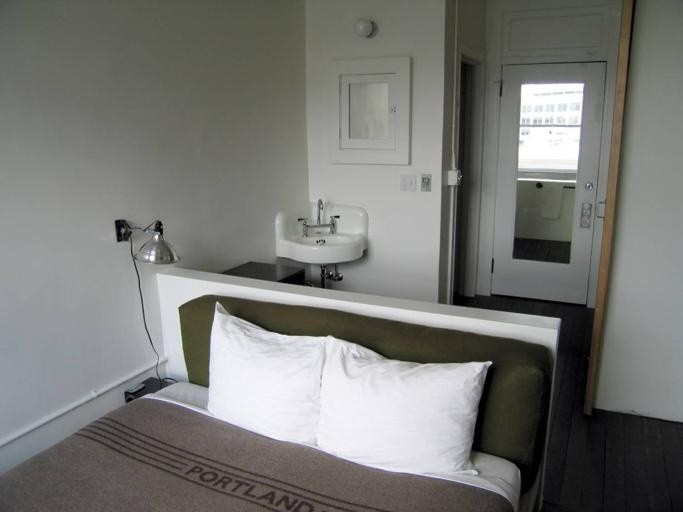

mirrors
left=329, top=56, right=414, bottom=166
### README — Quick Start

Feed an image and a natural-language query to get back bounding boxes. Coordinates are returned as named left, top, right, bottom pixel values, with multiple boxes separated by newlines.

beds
left=1, top=268, right=561, bottom=511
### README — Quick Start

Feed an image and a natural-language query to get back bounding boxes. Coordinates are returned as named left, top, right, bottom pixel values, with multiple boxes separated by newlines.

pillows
left=207, top=298, right=332, bottom=450
left=317, top=333, right=494, bottom=477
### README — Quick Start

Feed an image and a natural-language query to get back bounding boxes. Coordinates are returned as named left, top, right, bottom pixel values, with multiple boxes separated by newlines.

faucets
left=316, top=198, right=324, bottom=224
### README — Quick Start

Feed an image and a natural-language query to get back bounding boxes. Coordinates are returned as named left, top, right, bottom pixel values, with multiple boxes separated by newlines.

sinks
left=280, top=228, right=364, bottom=265
